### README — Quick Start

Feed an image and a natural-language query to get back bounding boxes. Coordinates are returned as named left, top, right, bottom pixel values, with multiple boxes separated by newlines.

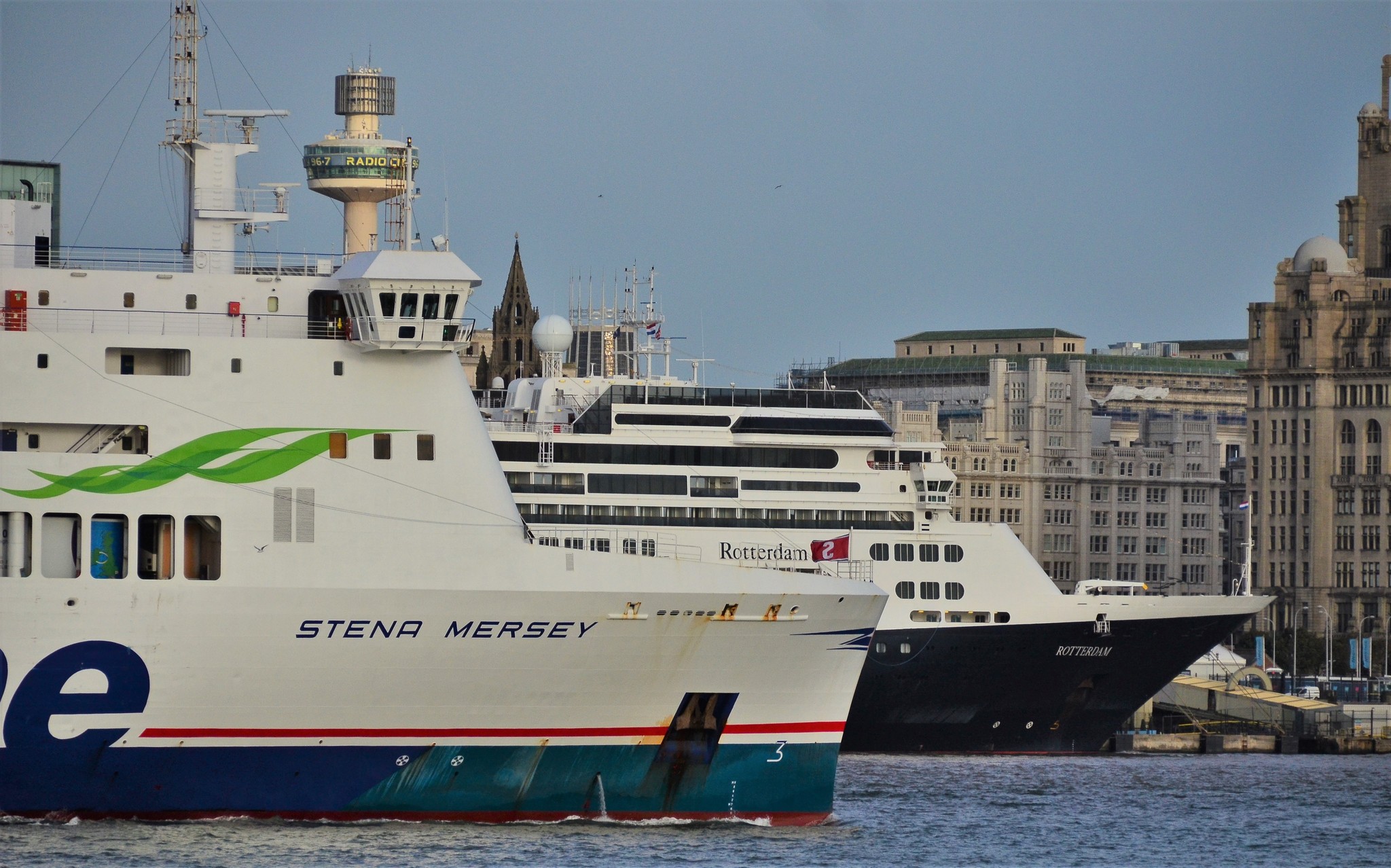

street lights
left=1319, top=611, right=1332, bottom=676
left=1359, top=616, right=1375, bottom=678
left=1294, top=607, right=1309, bottom=692
left=1263, top=618, right=1276, bottom=667
left=1317, top=605, right=1328, bottom=689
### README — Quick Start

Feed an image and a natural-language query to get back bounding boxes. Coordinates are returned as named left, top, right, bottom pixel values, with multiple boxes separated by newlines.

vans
left=1286, top=686, right=1320, bottom=701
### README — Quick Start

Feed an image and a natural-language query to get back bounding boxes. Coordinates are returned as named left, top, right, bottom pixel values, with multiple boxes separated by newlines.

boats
left=1, top=0, right=890, bottom=827
left=456, top=234, right=1281, bottom=761
left=1074, top=578, right=1246, bottom=683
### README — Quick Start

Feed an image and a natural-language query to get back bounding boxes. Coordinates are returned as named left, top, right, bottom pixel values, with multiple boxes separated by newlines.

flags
left=646, top=323, right=655, bottom=335
left=1362, top=638, right=1370, bottom=668
left=1350, top=639, right=1356, bottom=669
left=655, top=325, right=661, bottom=340
left=1239, top=501, right=1249, bottom=510
left=810, top=534, right=850, bottom=563
left=1256, top=637, right=1262, bottom=667
